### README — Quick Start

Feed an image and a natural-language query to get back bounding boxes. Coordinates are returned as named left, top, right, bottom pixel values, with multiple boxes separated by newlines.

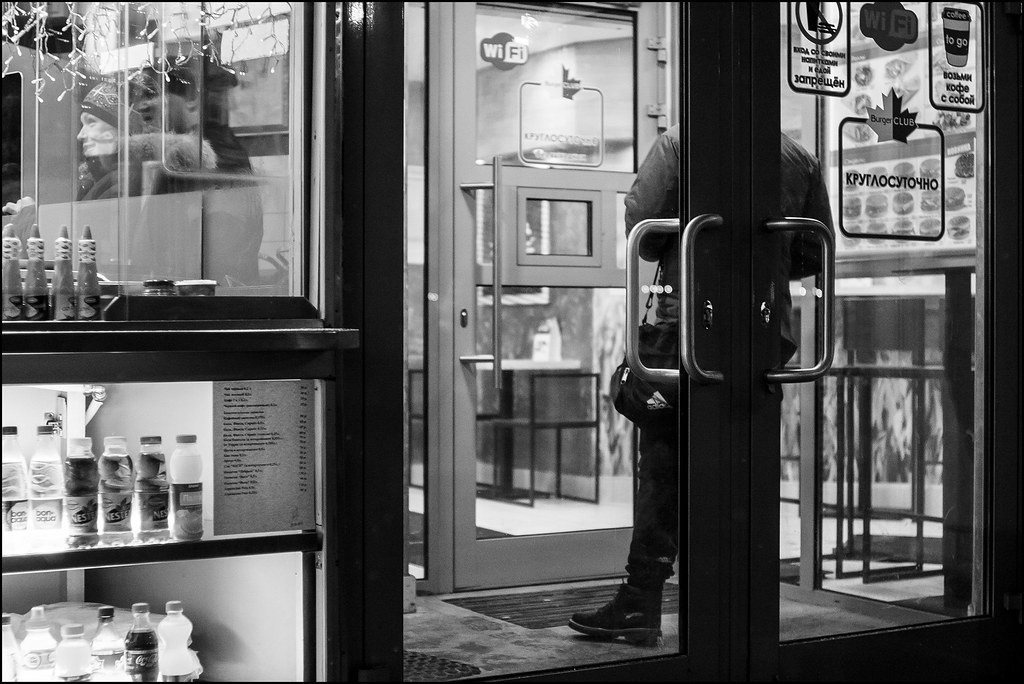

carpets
left=826, top=532, right=944, bottom=562
left=445, top=582, right=679, bottom=628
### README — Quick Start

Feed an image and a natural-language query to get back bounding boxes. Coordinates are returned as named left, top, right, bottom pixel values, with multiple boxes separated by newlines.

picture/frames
left=476, top=159, right=551, bottom=308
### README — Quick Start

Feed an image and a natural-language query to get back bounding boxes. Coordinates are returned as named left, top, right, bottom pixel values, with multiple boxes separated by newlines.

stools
left=780, top=298, right=929, bottom=576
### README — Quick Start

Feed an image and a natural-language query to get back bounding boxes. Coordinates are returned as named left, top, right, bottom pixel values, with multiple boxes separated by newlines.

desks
left=475, top=358, right=583, bottom=498
left=838, top=245, right=976, bottom=618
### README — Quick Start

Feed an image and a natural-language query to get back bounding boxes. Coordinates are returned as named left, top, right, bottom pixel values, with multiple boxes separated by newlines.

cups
left=174, top=280, right=220, bottom=295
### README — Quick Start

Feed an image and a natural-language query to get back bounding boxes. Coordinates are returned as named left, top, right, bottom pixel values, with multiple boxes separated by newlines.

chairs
left=490, top=370, right=602, bottom=508
left=407, top=367, right=499, bottom=498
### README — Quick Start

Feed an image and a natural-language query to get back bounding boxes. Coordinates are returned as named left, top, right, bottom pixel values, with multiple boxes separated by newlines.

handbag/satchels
left=610, top=322, right=681, bottom=429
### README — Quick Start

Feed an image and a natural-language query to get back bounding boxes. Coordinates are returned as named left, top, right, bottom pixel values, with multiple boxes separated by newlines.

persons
left=568, top=124, right=834, bottom=643
left=67, top=59, right=264, bottom=291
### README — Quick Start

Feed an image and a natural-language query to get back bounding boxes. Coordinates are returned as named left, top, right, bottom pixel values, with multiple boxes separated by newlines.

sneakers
left=567, top=581, right=661, bottom=648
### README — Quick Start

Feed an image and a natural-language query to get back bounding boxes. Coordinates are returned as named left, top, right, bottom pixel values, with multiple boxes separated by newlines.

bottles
left=2, top=224, right=23, bottom=319
left=63, top=438, right=100, bottom=549
left=124, top=602, right=160, bottom=682
left=134, top=435, right=171, bottom=544
left=2, top=426, right=28, bottom=558
left=2, top=615, right=19, bottom=682
left=55, top=623, right=92, bottom=682
left=17, top=606, right=57, bottom=682
left=97, top=437, right=134, bottom=546
left=28, top=426, right=66, bottom=555
left=51, top=226, right=76, bottom=321
left=76, top=225, right=102, bottom=320
left=25, top=223, right=50, bottom=320
left=169, top=434, right=204, bottom=541
left=89, top=605, right=127, bottom=681
left=157, top=600, right=196, bottom=682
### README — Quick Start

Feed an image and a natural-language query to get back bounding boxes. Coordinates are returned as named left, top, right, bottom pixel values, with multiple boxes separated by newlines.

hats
left=81, top=80, right=119, bottom=126
left=131, top=55, right=238, bottom=99
